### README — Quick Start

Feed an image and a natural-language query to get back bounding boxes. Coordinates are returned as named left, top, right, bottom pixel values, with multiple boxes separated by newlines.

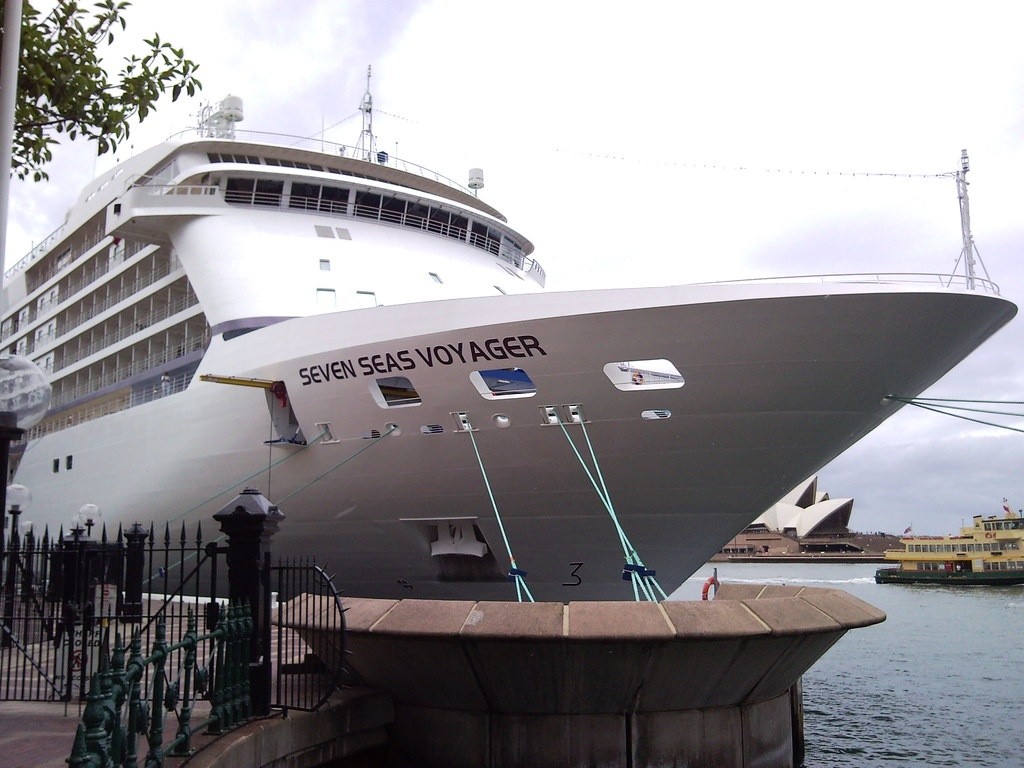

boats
left=874, top=498, right=1024, bottom=587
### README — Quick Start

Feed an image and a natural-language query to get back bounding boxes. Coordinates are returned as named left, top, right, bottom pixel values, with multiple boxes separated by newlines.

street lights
left=1, top=352, right=52, bottom=556
left=0, top=482, right=32, bottom=649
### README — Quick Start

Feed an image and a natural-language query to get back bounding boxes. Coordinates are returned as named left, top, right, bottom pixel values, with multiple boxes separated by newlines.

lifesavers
left=993, top=532, right=996, bottom=537
left=702, top=577, right=720, bottom=601
left=985, top=533, right=992, bottom=538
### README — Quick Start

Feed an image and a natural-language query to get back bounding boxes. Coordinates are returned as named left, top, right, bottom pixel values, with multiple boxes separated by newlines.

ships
left=1, top=67, right=1018, bottom=605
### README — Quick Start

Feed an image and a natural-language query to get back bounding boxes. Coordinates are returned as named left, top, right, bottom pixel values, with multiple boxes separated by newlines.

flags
left=904, top=526, right=911, bottom=533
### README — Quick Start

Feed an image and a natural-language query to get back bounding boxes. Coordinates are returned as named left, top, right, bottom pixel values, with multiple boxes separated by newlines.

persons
left=956, top=564, right=961, bottom=572
